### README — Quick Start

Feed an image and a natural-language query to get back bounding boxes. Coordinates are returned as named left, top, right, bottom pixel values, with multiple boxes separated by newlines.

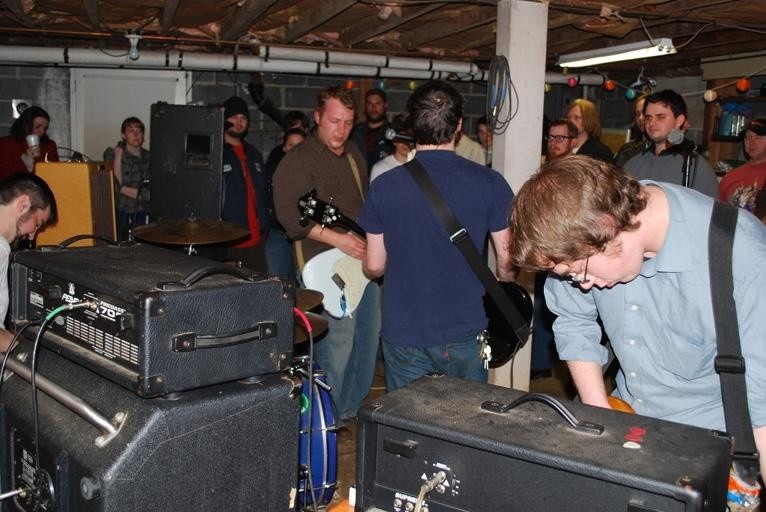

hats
left=739, top=119, right=766, bottom=136
left=225, top=97, right=249, bottom=119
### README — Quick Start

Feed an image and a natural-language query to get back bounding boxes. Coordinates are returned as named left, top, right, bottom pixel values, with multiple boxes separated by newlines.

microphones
left=668, top=128, right=697, bottom=152
left=386, top=128, right=416, bottom=141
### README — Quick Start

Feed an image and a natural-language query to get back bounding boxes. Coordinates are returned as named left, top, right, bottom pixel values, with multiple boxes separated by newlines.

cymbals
left=130, top=218, right=252, bottom=245
left=294, top=310, right=330, bottom=344
left=295, top=288, right=324, bottom=313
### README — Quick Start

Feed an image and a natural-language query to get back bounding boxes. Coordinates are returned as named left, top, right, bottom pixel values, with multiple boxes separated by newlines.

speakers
left=150, top=103, right=226, bottom=224
left=355, top=372, right=733, bottom=511
left=1, top=336, right=303, bottom=511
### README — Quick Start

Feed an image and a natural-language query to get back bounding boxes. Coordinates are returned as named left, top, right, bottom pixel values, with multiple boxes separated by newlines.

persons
left=103, top=116, right=150, bottom=238
left=542, top=98, right=614, bottom=167
left=508, top=156, right=765, bottom=480
left=611, top=90, right=718, bottom=200
left=221, top=79, right=389, bottom=442
left=361, top=80, right=516, bottom=392
left=1, top=106, right=60, bottom=181
left=717, top=118, right=763, bottom=222
left=0, top=170, right=61, bottom=354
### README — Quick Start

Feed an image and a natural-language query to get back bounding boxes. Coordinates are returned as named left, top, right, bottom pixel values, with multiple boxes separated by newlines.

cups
left=26, top=134, right=40, bottom=157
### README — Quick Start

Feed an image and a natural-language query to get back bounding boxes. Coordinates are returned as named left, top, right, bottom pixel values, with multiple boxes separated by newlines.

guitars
left=299, top=188, right=533, bottom=369
left=301, top=149, right=417, bottom=319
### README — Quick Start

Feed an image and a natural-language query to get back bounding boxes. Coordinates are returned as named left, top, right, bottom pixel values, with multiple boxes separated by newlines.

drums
left=292, top=353, right=337, bottom=512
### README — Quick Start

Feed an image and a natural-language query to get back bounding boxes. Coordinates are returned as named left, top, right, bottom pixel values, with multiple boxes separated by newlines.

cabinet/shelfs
left=701, top=75, right=766, bottom=178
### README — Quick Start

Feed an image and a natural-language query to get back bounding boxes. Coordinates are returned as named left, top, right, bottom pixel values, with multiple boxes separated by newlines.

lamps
left=558, top=37, right=677, bottom=67
left=125, top=35, right=143, bottom=60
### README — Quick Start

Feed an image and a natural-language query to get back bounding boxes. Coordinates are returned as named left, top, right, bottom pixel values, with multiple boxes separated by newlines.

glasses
left=544, top=135, right=569, bottom=143
left=571, top=258, right=591, bottom=283
